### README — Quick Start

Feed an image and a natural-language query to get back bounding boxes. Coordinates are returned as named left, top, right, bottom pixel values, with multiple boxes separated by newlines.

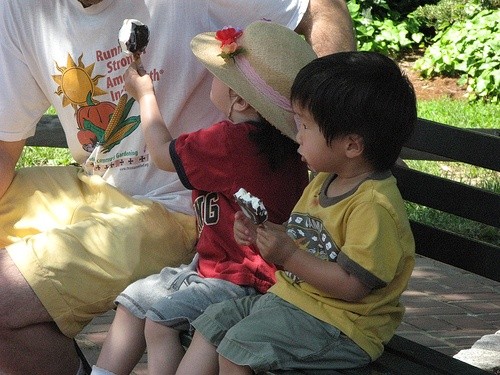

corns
left=83, top=93, right=140, bottom=152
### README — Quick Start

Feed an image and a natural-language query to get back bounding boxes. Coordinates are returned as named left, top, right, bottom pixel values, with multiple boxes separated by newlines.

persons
left=0, top=0, right=356, bottom=375
left=91, top=20, right=320, bottom=375
left=175, top=50, right=418, bottom=375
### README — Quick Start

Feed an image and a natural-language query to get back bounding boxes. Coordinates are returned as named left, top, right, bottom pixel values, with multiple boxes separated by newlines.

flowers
left=215, top=25, right=245, bottom=64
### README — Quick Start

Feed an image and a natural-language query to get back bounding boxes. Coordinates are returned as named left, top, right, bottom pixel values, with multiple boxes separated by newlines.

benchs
left=180, top=117, right=500, bottom=375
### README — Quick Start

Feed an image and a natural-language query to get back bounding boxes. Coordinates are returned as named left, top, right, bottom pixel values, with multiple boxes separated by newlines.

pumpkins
left=75, top=91, right=118, bottom=145
